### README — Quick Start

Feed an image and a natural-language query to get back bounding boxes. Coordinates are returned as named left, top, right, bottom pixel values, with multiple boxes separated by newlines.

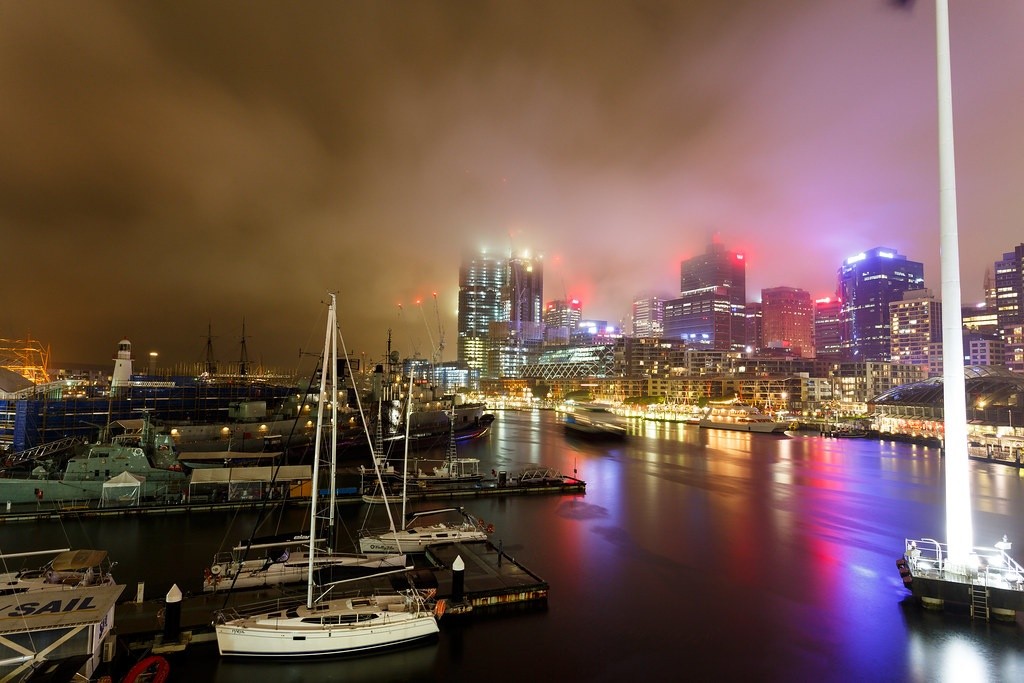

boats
left=564, top=406, right=628, bottom=437
left=820, top=426, right=870, bottom=439
left=699, top=407, right=791, bottom=434
left=0, top=315, right=495, bottom=513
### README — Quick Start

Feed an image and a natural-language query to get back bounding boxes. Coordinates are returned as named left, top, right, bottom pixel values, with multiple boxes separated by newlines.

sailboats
left=358, top=362, right=488, bottom=555
left=212, top=290, right=441, bottom=660
left=201, top=338, right=407, bottom=594
